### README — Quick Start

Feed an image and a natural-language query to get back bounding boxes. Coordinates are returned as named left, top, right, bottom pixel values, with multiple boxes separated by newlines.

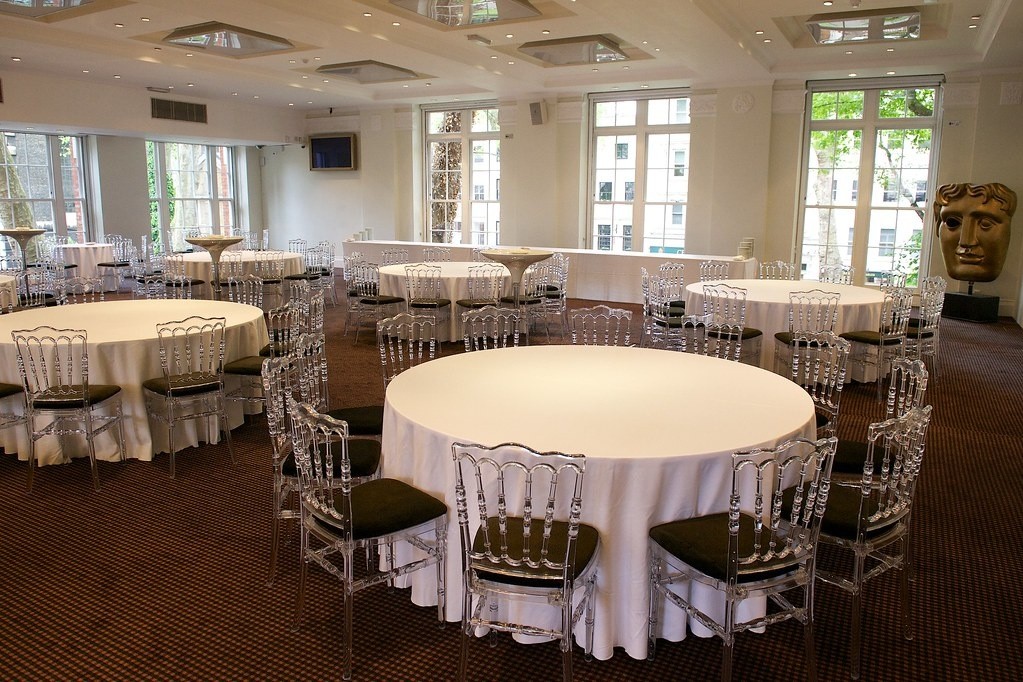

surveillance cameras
left=255, top=145, right=263, bottom=149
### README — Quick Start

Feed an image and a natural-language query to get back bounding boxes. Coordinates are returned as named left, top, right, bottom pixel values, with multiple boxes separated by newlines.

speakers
left=529, top=80, right=548, bottom=125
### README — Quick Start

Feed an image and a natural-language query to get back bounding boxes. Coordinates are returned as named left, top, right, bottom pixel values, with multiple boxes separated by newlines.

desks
left=1, top=300, right=272, bottom=463
left=381, top=345, right=813, bottom=664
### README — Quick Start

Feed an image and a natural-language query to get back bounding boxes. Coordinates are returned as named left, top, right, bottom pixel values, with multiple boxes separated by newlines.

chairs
left=0, top=385, right=34, bottom=489
left=9, top=326, right=123, bottom=494
left=141, top=316, right=235, bottom=479
left=833, top=361, right=929, bottom=482
left=791, top=333, right=846, bottom=430
left=0, top=229, right=942, bottom=387
left=299, top=285, right=330, bottom=415
left=297, top=333, right=383, bottom=441
left=453, top=440, right=600, bottom=681
left=291, top=403, right=447, bottom=679
left=777, top=410, right=930, bottom=682
left=648, top=436, right=838, bottom=681
left=259, top=360, right=380, bottom=573
left=223, top=303, right=300, bottom=437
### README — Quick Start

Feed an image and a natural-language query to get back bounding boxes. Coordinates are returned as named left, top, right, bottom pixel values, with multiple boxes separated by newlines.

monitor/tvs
left=308, top=134, right=356, bottom=171
